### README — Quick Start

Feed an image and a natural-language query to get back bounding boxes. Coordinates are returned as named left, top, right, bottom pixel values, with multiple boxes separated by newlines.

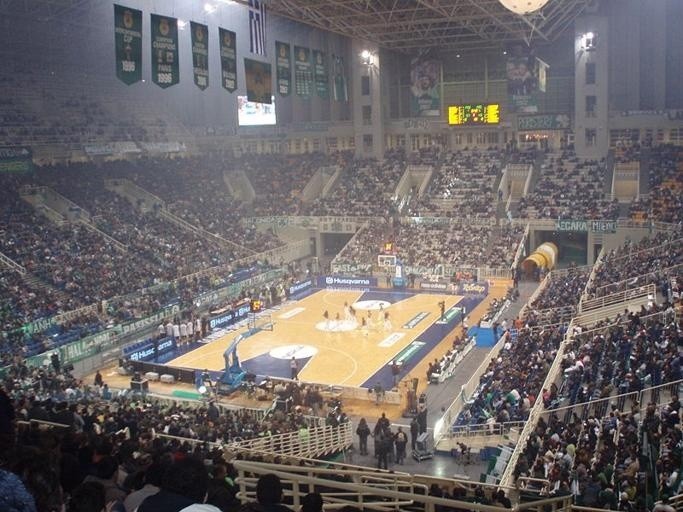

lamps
left=585, top=32, right=596, bottom=49
left=360, top=48, right=374, bottom=66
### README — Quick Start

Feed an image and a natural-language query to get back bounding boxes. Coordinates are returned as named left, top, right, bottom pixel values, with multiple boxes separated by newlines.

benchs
left=517, top=149, right=612, bottom=220
left=310, top=150, right=505, bottom=219
left=631, top=161, right=683, bottom=219
left=550, top=385, right=683, bottom=423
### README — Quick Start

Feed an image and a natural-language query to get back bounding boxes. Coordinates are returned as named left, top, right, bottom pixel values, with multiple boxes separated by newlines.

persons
left=1, top=49, right=682, bottom=511
